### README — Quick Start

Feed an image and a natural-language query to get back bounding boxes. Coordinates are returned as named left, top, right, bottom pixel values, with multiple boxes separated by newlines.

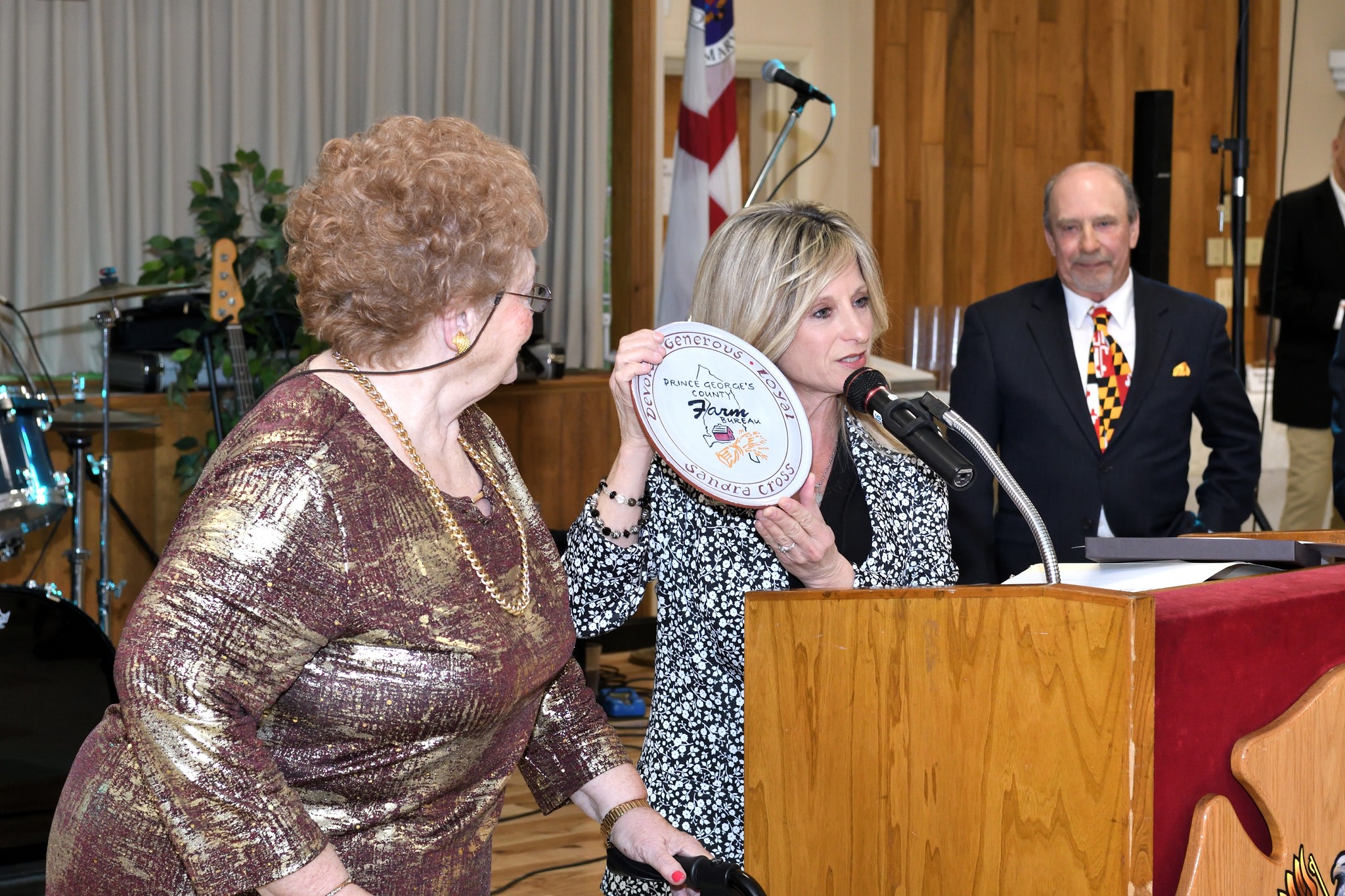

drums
left=0, top=385, right=73, bottom=560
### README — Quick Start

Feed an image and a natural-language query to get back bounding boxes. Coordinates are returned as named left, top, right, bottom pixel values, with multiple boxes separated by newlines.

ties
left=1084, top=306, right=1133, bottom=453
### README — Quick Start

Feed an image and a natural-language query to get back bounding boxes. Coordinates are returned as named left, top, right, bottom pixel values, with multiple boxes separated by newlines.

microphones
left=843, top=367, right=977, bottom=492
left=761, top=58, right=834, bottom=107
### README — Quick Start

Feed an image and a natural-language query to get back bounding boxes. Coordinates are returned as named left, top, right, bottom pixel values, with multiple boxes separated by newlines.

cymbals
left=16, top=267, right=205, bottom=314
left=43, top=371, right=159, bottom=430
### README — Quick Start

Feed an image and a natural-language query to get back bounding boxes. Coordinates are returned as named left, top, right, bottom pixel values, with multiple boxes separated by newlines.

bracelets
left=599, top=476, right=648, bottom=509
left=590, top=487, right=648, bottom=540
left=594, top=794, right=652, bottom=847
left=318, top=875, right=355, bottom=896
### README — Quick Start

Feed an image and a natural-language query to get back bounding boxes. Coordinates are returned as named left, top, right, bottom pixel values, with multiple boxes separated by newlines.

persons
left=552, top=205, right=966, bottom=896
left=42, top=116, right=726, bottom=896
left=1253, top=118, right=1344, bottom=531
left=937, top=160, right=1267, bottom=586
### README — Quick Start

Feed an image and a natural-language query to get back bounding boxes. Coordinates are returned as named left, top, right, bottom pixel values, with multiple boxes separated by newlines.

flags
left=651, top=0, right=750, bottom=337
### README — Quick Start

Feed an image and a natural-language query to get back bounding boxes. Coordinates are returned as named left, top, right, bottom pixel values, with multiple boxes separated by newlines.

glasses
left=499, top=284, right=552, bottom=314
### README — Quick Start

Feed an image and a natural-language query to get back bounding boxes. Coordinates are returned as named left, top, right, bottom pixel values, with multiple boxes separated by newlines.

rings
left=778, top=541, right=796, bottom=553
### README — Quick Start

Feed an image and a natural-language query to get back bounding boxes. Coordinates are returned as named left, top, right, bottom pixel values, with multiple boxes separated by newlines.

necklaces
left=327, top=343, right=534, bottom=618
left=813, top=443, right=836, bottom=504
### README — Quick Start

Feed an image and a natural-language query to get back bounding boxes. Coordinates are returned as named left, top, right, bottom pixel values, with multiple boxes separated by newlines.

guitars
left=212, top=237, right=254, bottom=417
